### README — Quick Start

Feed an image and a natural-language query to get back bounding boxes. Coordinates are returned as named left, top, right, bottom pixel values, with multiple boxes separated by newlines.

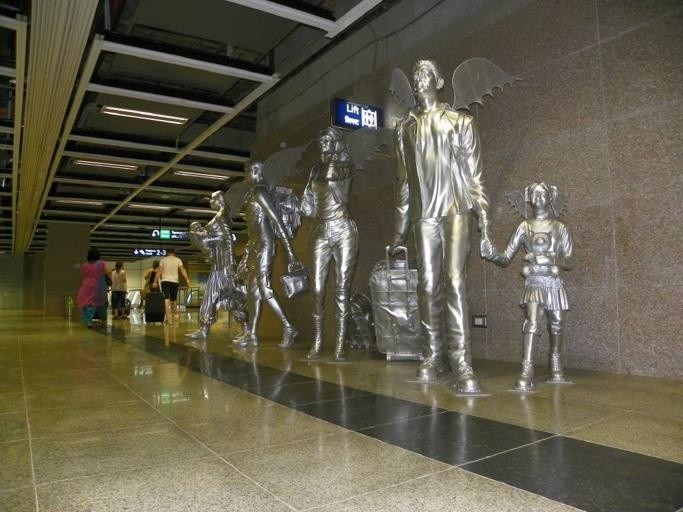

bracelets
left=185, top=280, right=189, bottom=283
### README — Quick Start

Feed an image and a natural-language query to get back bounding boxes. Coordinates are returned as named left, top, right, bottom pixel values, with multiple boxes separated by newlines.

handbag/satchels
left=141, top=284, right=150, bottom=301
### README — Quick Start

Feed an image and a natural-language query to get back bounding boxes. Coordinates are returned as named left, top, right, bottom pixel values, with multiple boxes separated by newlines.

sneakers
left=86, top=314, right=176, bottom=329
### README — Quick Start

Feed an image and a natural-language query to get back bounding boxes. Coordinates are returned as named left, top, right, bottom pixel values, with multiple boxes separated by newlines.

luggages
left=145, top=287, right=166, bottom=323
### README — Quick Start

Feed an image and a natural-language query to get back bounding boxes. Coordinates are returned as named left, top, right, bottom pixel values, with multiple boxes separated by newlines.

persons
left=77, top=247, right=112, bottom=330
left=184, top=189, right=250, bottom=345
left=484, top=181, right=572, bottom=392
left=145, top=259, right=161, bottom=292
left=231, top=157, right=297, bottom=349
left=388, top=67, right=494, bottom=396
left=152, top=248, right=190, bottom=323
left=298, top=126, right=360, bottom=363
left=189, top=221, right=205, bottom=232
left=112, top=261, right=128, bottom=319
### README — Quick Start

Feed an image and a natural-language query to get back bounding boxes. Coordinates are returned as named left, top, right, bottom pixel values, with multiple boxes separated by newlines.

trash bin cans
left=64, top=295, right=80, bottom=317
